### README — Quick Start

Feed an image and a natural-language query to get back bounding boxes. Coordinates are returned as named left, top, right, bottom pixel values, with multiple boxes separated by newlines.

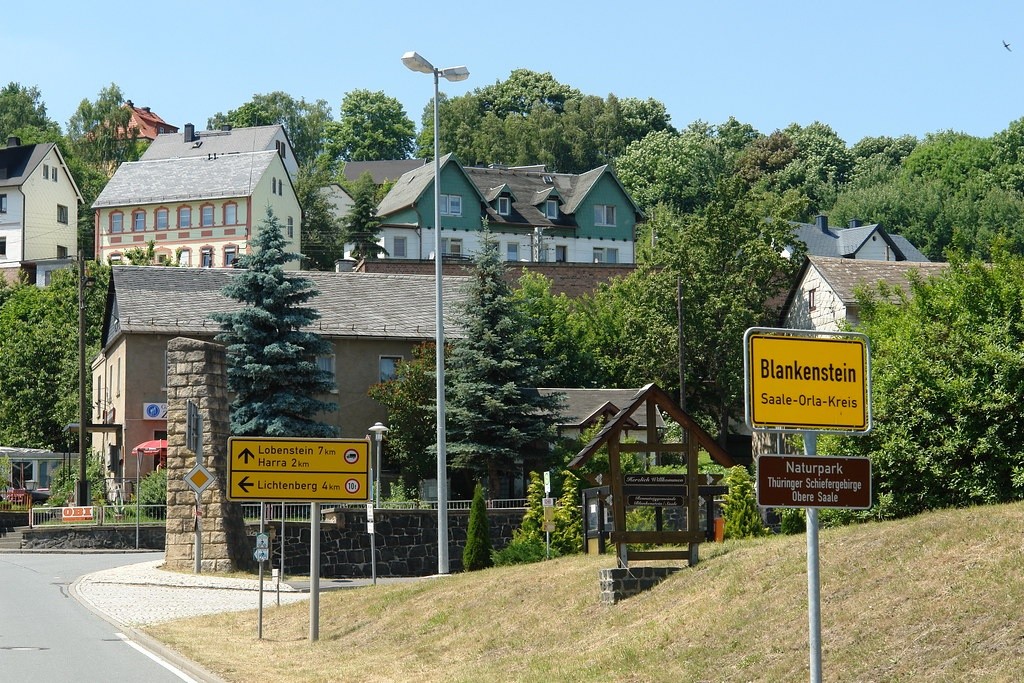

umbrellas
left=132, top=439, right=168, bottom=463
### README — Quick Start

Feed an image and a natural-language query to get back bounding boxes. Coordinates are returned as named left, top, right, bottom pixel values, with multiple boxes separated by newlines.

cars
left=0, top=481, right=51, bottom=508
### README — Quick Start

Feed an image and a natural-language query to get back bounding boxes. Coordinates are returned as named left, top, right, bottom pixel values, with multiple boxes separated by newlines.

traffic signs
left=227, top=437, right=370, bottom=505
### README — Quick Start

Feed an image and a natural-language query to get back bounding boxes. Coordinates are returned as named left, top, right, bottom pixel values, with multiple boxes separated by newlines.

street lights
left=370, top=421, right=390, bottom=510
left=75, top=276, right=95, bottom=506
left=403, top=51, right=469, bottom=573
left=62, top=439, right=74, bottom=484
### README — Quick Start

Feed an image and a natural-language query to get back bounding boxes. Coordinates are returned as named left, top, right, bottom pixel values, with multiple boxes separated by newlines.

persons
left=156, top=457, right=166, bottom=473
